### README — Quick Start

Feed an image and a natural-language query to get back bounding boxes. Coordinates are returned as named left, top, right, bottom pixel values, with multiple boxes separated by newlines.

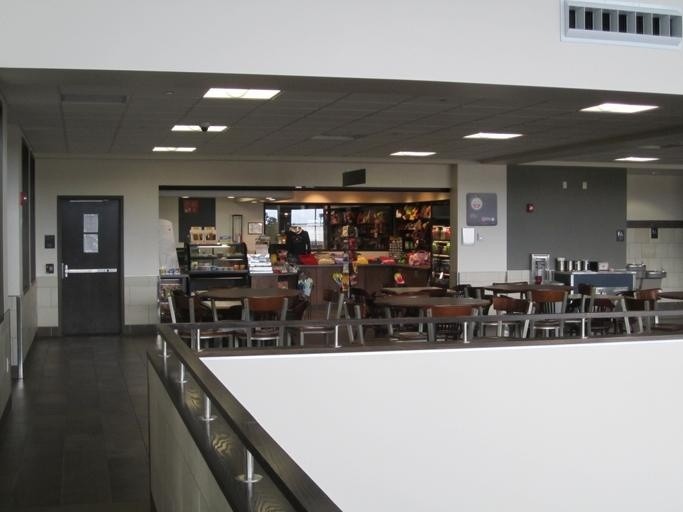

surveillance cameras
left=199, top=122, right=210, bottom=132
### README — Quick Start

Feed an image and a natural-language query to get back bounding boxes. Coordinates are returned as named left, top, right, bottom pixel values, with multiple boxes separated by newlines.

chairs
left=166, top=272, right=664, bottom=350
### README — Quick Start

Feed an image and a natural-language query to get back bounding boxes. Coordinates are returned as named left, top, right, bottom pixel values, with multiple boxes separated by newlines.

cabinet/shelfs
left=182, top=241, right=251, bottom=320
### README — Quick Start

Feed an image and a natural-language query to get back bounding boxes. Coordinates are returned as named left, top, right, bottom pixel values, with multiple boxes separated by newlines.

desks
left=615, top=290, right=682, bottom=299
